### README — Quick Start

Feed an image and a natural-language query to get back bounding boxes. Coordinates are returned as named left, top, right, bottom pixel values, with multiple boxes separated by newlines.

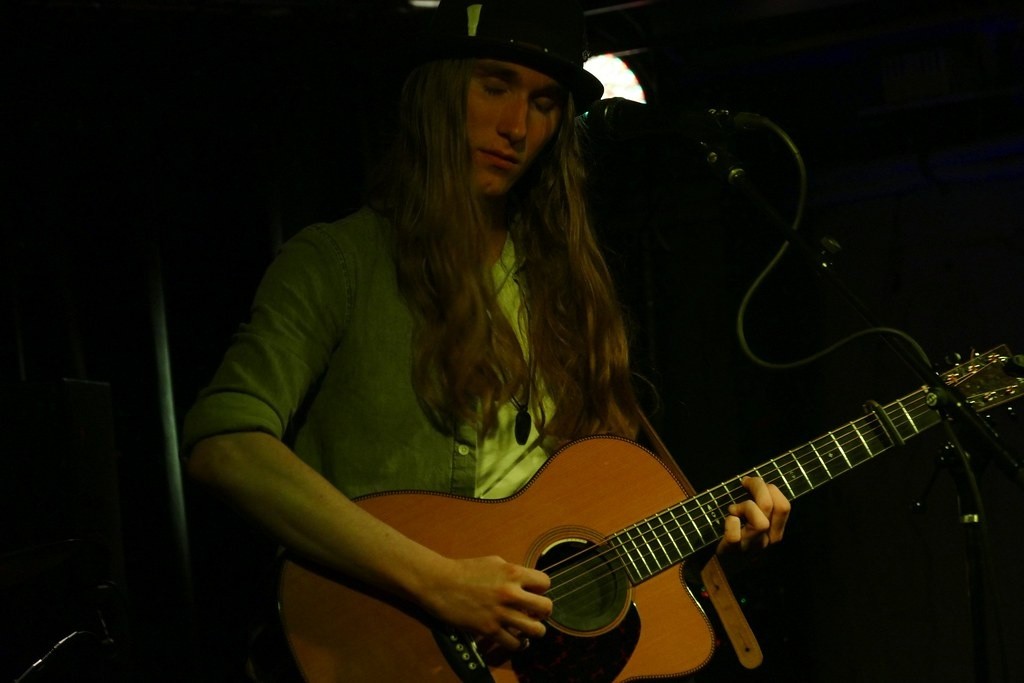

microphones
left=587, top=96, right=760, bottom=137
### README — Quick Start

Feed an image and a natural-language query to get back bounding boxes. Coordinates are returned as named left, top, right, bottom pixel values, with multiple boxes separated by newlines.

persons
left=181, top=1, right=793, bottom=649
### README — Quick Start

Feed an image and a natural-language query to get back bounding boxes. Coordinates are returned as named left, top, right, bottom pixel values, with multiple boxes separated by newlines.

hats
left=414, top=0, right=604, bottom=102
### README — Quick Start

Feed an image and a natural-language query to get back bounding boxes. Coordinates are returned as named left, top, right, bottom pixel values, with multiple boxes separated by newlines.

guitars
left=248, top=339, right=1024, bottom=682
